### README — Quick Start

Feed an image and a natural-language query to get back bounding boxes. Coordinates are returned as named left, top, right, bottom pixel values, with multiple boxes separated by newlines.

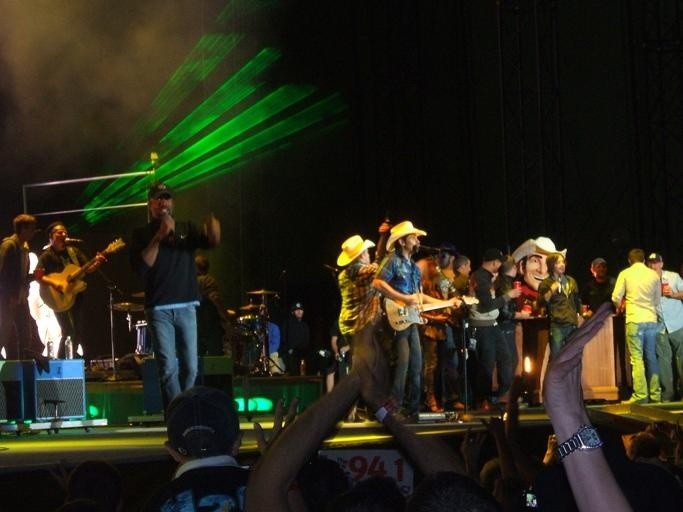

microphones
left=414, top=244, right=440, bottom=253
left=557, top=274, right=562, bottom=294
left=160, top=209, right=175, bottom=242
left=64, top=237, right=83, bottom=245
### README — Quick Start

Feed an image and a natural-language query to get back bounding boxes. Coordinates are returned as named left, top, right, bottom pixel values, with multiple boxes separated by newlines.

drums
left=230, top=325, right=262, bottom=373
left=133, top=320, right=156, bottom=358
left=237, top=314, right=269, bottom=332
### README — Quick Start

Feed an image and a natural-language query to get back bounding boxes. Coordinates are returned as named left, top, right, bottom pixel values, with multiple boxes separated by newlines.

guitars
left=382, top=293, right=480, bottom=334
left=40, top=238, right=127, bottom=312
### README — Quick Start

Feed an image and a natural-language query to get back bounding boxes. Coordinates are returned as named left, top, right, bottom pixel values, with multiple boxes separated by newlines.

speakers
left=0, top=359, right=86, bottom=423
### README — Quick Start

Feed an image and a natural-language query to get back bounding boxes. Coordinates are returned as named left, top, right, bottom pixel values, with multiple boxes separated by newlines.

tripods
left=232, top=294, right=284, bottom=377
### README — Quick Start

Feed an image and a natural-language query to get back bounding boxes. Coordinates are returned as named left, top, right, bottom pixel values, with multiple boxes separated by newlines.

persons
left=1, top=182, right=683, bottom=512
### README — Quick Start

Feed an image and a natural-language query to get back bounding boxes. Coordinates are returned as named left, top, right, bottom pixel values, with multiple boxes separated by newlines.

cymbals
left=238, top=304, right=263, bottom=311
left=133, top=292, right=145, bottom=297
left=110, top=302, right=144, bottom=312
left=246, top=290, right=276, bottom=295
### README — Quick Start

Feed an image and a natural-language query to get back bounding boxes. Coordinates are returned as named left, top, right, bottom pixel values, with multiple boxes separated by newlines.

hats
left=147, top=182, right=174, bottom=200
left=163, top=386, right=240, bottom=461
left=481, top=247, right=508, bottom=262
left=386, top=221, right=427, bottom=252
left=336, top=235, right=376, bottom=267
left=646, top=252, right=662, bottom=263
left=289, top=302, right=304, bottom=311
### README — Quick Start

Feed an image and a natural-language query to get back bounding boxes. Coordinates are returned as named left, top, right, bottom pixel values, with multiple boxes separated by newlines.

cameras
left=520, top=489, right=542, bottom=512
